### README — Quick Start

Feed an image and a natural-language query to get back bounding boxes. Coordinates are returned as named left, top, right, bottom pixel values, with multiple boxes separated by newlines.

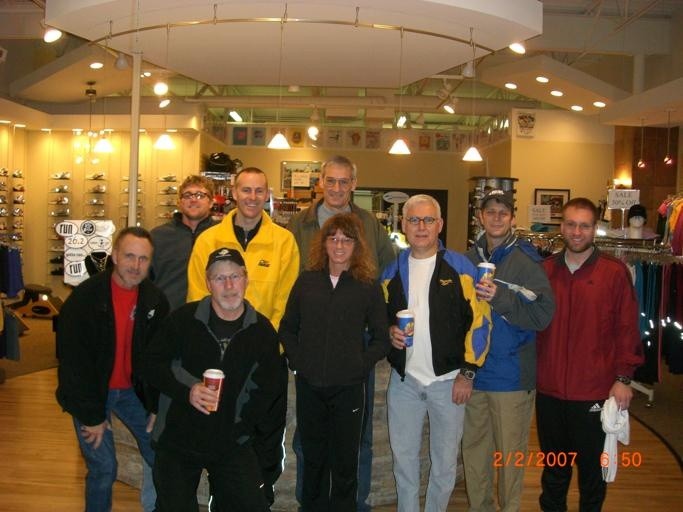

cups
left=203, top=369, right=225, bottom=412
left=477, top=263, right=496, bottom=301
left=396, top=310, right=415, bottom=348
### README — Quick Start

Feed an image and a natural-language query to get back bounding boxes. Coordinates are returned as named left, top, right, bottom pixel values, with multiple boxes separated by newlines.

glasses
left=406, top=217, right=437, bottom=224
left=209, top=272, right=244, bottom=281
left=564, top=220, right=593, bottom=229
left=181, top=191, right=212, bottom=199
left=323, top=177, right=353, bottom=186
left=327, top=237, right=354, bottom=244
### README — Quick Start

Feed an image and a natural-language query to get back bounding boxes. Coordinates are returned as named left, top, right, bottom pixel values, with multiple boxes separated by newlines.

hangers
left=546, top=240, right=672, bottom=409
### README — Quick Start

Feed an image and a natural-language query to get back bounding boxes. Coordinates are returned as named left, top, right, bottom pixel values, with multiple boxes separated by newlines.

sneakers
left=159, top=175, right=176, bottom=182
left=0, top=207, right=8, bottom=216
left=0, top=222, right=9, bottom=230
left=49, top=255, right=64, bottom=264
left=0, top=195, right=8, bottom=204
left=12, top=169, right=22, bottom=178
left=12, top=218, right=24, bottom=229
left=50, top=171, right=70, bottom=179
left=160, top=186, right=177, bottom=194
left=8, top=232, right=22, bottom=241
left=123, top=174, right=141, bottom=180
left=86, top=170, right=105, bottom=180
left=125, top=187, right=141, bottom=193
left=88, top=209, right=104, bottom=217
left=123, top=200, right=142, bottom=207
left=121, top=211, right=144, bottom=219
left=0, top=181, right=7, bottom=191
left=14, top=194, right=25, bottom=204
left=12, top=208, right=24, bottom=217
left=159, top=209, right=178, bottom=218
left=51, top=267, right=64, bottom=275
left=160, top=199, right=177, bottom=206
left=0, top=168, right=10, bottom=177
left=49, top=208, right=70, bottom=217
left=87, top=198, right=104, bottom=205
left=13, top=184, right=25, bottom=192
left=89, top=184, right=105, bottom=193
left=51, top=244, right=64, bottom=251
left=50, top=185, right=69, bottom=192
left=48, top=196, right=69, bottom=205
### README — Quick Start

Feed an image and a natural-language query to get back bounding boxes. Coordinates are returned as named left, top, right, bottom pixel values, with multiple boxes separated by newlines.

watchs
left=614, top=375, right=632, bottom=387
left=457, top=368, right=476, bottom=382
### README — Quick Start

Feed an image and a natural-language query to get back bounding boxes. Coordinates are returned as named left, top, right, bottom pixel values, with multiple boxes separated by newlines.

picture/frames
left=281, top=160, right=321, bottom=191
left=534, top=188, right=571, bottom=226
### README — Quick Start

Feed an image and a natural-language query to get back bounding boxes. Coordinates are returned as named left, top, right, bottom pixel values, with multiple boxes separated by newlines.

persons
left=148, top=176, right=221, bottom=315
left=461, top=190, right=557, bottom=511
left=278, top=212, right=392, bottom=512
left=624, top=204, right=659, bottom=239
left=286, top=156, right=397, bottom=512
left=144, top=247, right=288, bottom=512
left=540, top=198, right=645, bottom=512
left=187, top=167, right=300, bottom=356
left=379, top=195, right=493, bottom=508
left=55, top=227, right=171, bottom=512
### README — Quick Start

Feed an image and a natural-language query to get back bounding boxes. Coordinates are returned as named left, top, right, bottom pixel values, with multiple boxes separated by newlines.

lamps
left=307, top=124, right=320, bottom=135
left=664, top=111, right=672, bottom=165
left=550, top=90, right=564, bottom=97
left=153, top=24, right=176, bottom=150
left=462, top=64, right=475, bottom=78
left=388, top=28, right=412, bottom=155
left=570, top=105, right=584, bottom=111
left=415, top=115, right=425, bottom=126
left=462, top=42, right=482, bottom=162
left=637, top=118, right=645, bottom=168
left=92, top=38, right=114, bottom=153
left=159, top=96, right=170, bottom=108
left=39, top=19, right=62, bottom=43
left=113, top=56, right=129, bottom=71
left=504, top=81, right=519, bottom=90
left=154, top=80, right=168, bottom=95
left=443, top=100, right=457, bottom=114
left=89, top=58, right=104, bottom=70
left=508, top=42, right=526, bottom=54
left=268, top=21, right=291, bottom=150
left=592, top=101, right=607, bottom=109
left=536, top=76, right=549, bottom=84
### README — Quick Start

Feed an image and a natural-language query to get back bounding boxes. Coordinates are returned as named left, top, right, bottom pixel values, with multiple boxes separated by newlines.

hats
left=627, top=204, right=648, bottom=227
left=480, top=189, right=514, bottom=213
left=205, top=247, right=245, bottom=271
